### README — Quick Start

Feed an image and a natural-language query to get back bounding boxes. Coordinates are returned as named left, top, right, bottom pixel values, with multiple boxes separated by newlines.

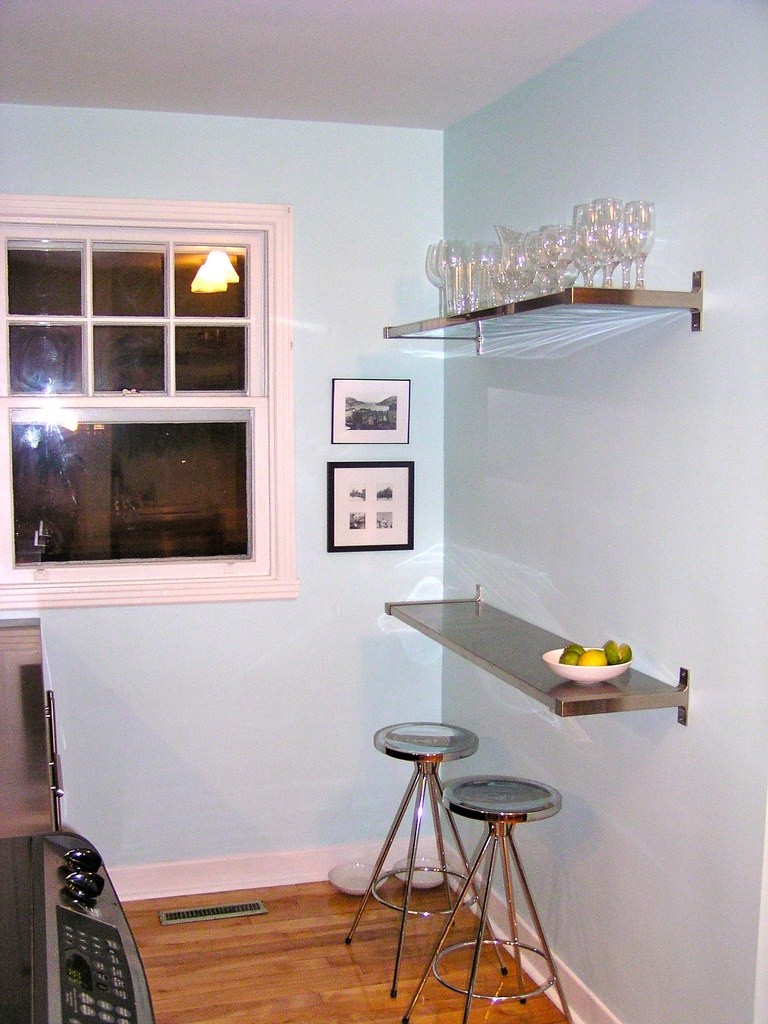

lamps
left=191, top=251, right=240, bottom=293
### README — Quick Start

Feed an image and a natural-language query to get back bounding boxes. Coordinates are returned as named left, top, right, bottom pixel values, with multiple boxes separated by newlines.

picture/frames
left=327, top=461, right=414, bottom=552
left=331, top=378, right=411, bottom=444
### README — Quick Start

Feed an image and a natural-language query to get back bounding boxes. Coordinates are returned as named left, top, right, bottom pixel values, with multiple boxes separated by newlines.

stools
left=346, top=722, right=509, bottom=997
left=403, top=775, right=574, bottom=1024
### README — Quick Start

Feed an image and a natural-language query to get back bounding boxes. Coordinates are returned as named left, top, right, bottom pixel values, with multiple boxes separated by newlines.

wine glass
left=426, top=198, right=656, bottom=318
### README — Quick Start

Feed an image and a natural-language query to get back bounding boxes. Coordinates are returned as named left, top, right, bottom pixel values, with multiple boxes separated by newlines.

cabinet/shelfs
left=381, top=269, right=702, bottom=726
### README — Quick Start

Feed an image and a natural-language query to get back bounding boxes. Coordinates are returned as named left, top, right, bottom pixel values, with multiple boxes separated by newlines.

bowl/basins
left=394, top=858, right=452, bottom=888
left=542, top=648, right=632, bottom=685
left=328, top=862, right=388, bottom=895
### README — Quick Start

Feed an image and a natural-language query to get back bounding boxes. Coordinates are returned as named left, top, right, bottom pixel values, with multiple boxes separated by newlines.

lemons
left=559, top=639, right=634, bottom=668
